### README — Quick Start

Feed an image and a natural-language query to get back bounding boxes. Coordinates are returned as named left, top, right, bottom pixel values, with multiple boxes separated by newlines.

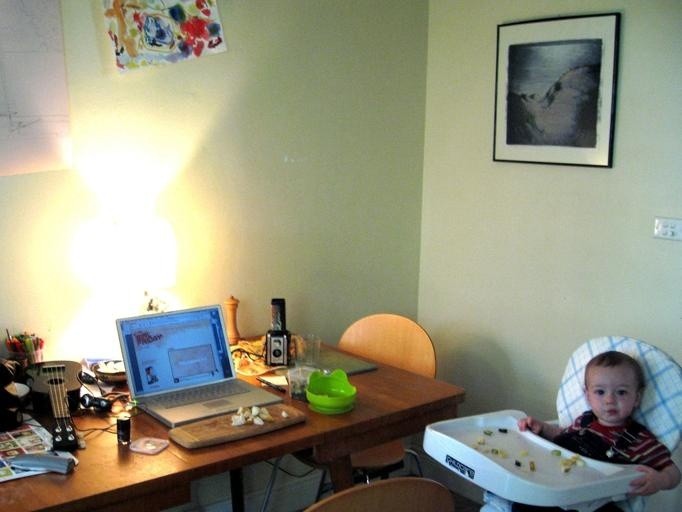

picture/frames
left=494, top=11, right=620, bottom=169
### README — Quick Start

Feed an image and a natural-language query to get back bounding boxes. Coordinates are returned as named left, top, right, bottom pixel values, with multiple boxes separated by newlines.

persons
left=145, top=366, right=159, bottom=385
left=509, top=351, right=681, bottom=512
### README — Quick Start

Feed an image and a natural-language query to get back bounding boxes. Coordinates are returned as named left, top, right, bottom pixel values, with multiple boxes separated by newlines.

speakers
left=265, top=329, right=291, bottom=365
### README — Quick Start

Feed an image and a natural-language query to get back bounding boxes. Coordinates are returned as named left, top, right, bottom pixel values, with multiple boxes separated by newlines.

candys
left=471, top=428, right=585, bottom=473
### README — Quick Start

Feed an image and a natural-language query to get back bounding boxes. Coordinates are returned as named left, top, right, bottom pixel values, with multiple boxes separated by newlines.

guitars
left=25, top=361, right=84, bottom=452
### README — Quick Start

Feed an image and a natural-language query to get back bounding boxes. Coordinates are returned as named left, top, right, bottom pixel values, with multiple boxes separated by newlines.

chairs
left=291, top=313, right=460, bottom=511
left=423, top=337, right=681, bottom=512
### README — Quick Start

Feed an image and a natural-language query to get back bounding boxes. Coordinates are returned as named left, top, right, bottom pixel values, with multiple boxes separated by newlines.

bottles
left=264, top=298, right=291, bottom=368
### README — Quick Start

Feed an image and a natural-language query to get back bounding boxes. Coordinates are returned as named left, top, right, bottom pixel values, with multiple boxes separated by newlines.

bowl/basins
left=90, top=358, right=128, bottom=382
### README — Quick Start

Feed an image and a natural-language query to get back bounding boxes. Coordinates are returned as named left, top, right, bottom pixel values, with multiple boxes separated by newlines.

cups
left=7, top=345, right=46, bottom=368
left=294, top=331, right=320, bottom=368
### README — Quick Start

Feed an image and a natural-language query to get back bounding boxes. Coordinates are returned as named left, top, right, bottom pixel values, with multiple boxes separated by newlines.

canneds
left=117, top=418, right=130, bottom=444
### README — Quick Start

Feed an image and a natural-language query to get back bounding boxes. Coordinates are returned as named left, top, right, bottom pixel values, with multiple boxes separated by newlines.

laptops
left=114, top=304, right=282, bottom=429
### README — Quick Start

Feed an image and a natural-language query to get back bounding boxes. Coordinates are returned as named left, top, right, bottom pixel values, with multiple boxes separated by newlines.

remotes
left=10, top=454, right=76, bottom=475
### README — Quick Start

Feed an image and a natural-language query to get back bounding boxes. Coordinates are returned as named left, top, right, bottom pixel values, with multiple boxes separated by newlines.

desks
left=0, top=340, right=464, bottom=510
